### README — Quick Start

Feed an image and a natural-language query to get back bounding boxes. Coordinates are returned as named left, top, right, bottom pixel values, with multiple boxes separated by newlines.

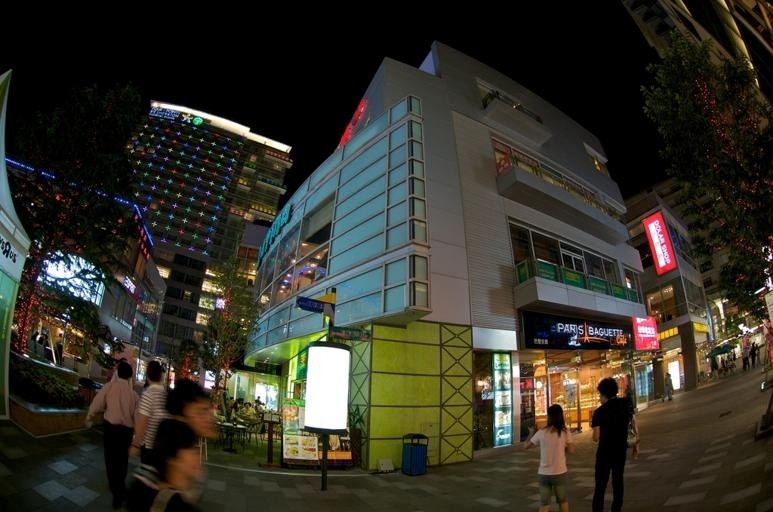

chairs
left=270, top=414, right=279, bottom=441
left=244, top=421, right=263, bottom=443
left=27, top=340, right=89, bottom=378
left=195, top=436, right=208, bottom=464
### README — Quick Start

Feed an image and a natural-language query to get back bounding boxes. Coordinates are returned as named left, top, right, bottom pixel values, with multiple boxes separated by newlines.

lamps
left=535, top=377, right=542, bottom=388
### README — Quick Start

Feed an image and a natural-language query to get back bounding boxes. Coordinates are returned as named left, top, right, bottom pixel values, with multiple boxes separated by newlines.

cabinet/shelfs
left=563, top=378, right=622, bottom=423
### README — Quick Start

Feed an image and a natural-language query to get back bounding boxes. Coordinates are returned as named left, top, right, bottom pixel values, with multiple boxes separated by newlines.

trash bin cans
left=402, top=433, right=429, bottom=476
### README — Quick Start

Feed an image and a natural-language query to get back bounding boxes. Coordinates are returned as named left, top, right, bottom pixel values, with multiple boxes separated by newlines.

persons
left=30, top=331, right=63, bottom=361
left=524, top=404, right=576, bottom=512
left=710, top=330, right=760, bottom=380
left=85, top=361, right=265, bottom=512
left=623, top=386, right=632, bottom=399
left=662, top=373, right=673, bottom=402
left=592, top=377, right=641, bottom=512
left=625, top=408, right=640, bottom=464
